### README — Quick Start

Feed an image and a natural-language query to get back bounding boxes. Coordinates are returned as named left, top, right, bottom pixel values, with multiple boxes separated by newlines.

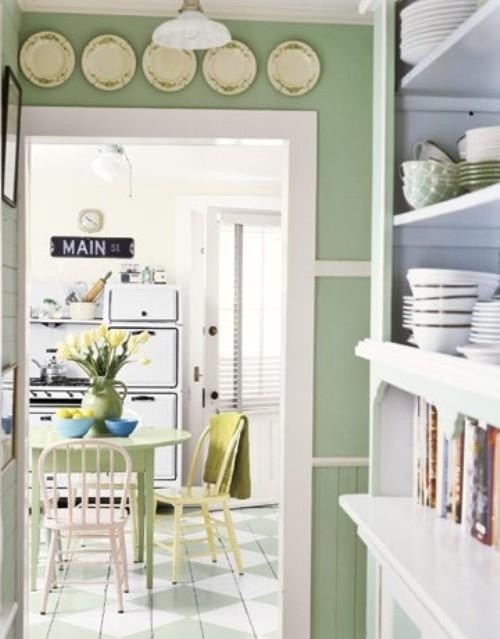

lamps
left=150, top=1, right=232, bottom=53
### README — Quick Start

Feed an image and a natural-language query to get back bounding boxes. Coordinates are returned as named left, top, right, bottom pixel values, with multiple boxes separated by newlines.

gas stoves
left=28, top=377, right=94, bottom=387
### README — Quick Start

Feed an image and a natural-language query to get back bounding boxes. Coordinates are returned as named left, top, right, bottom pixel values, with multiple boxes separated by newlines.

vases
left=82, top=374, right=127, bottom=436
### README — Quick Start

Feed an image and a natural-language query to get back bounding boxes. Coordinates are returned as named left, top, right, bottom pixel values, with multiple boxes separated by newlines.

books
left=413, top=395, right=500, bottom=552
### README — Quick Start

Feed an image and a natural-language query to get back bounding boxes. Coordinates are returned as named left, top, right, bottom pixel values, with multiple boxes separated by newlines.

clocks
left=77, top=208, right=104, bottom=232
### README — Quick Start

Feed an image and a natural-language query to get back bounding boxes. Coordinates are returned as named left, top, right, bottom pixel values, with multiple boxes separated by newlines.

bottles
left=142, top=265, right=151, bottom=284
left=154, top=266, right=167, bottom=284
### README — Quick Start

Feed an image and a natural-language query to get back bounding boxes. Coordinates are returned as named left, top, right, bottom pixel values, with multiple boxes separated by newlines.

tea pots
left=30, top=349, right=71, bottom=380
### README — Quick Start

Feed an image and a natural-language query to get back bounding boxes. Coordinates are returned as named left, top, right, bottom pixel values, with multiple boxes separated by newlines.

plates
left=266, top=40, right=320, bottom=96
left=142, top=38, right=198, bottom=92
left=453, top=124, right=499, bottom=191
left=81, top=32, right=138, bottom=92
left=201, top=40, right=257, bottom=96
left=400, top=0, right=476, bottom=64
left=19, top=30, right=76, bottom=89
left=402, top=265, right=500, bottom=365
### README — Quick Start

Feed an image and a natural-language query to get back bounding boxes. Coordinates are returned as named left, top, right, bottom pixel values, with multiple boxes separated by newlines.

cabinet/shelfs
left=339, top=0, right=500, bottom=639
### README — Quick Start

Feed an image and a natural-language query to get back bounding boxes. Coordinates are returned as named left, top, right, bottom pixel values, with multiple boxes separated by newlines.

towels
left=205, top=410, right=252, bottom=499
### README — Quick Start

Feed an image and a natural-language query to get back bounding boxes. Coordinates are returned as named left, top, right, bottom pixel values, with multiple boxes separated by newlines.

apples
left=56, top=409, right=94, bottom=418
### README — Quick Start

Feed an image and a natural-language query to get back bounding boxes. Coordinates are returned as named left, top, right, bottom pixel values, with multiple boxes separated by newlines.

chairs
left=36, top=441, right=132, bottom=614
left=145, top=410, right=254, bottom=585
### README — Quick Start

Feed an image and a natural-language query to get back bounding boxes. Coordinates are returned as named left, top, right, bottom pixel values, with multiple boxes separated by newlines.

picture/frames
left=0, top=63, right=23, bottom=210
left=2, top=361, right=19, bottom=476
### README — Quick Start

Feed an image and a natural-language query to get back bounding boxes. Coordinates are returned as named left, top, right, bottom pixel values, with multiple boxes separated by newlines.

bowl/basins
left=51, top=415, right=96, bottom=438
left=68, top=303, right=99, bottom=322
left=399, top=141, right=455, bottom=209
left=105, top=416, right=139, bottom=437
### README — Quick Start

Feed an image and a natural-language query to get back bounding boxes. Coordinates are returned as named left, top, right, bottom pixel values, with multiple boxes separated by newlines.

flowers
left=54, top=324, right=153, bottom=377
